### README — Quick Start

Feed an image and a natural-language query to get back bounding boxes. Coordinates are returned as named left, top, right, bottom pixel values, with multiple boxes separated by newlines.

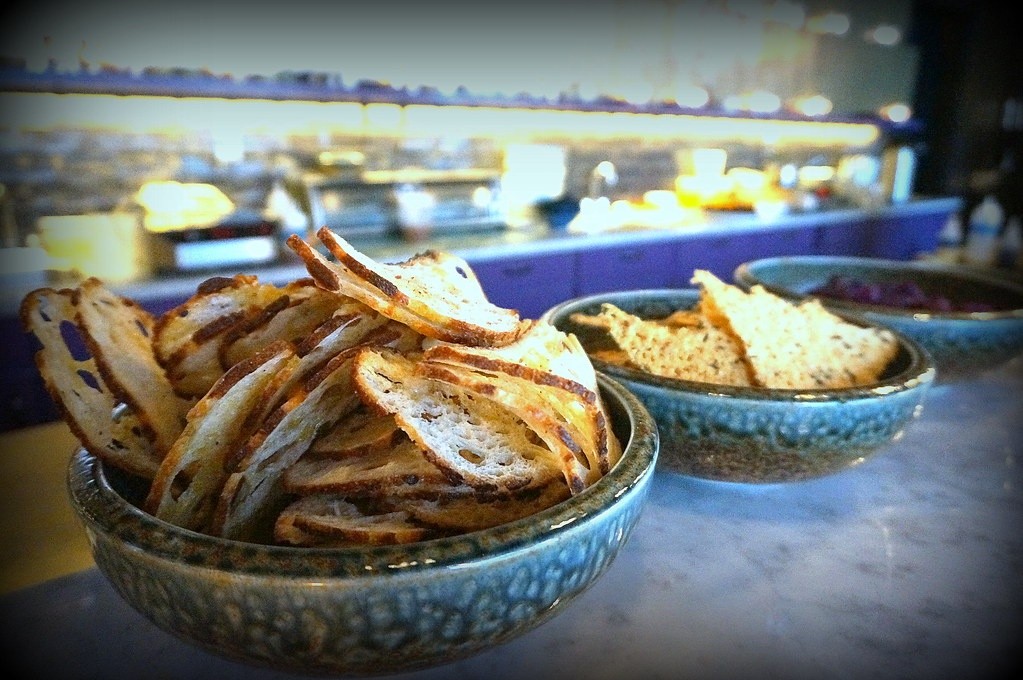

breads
left=18, top=227, right=622, bottom=552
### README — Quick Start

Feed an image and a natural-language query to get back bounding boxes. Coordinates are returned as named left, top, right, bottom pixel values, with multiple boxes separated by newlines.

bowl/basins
left=537, top=288, right=934, bottom=485
left=68, top=369, right=658, bottom=673
left=737, top=256, right=1020, bottom=377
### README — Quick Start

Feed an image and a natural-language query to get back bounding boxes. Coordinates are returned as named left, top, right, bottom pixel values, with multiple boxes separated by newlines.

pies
left=565, top=269, right=901, bottom=390
